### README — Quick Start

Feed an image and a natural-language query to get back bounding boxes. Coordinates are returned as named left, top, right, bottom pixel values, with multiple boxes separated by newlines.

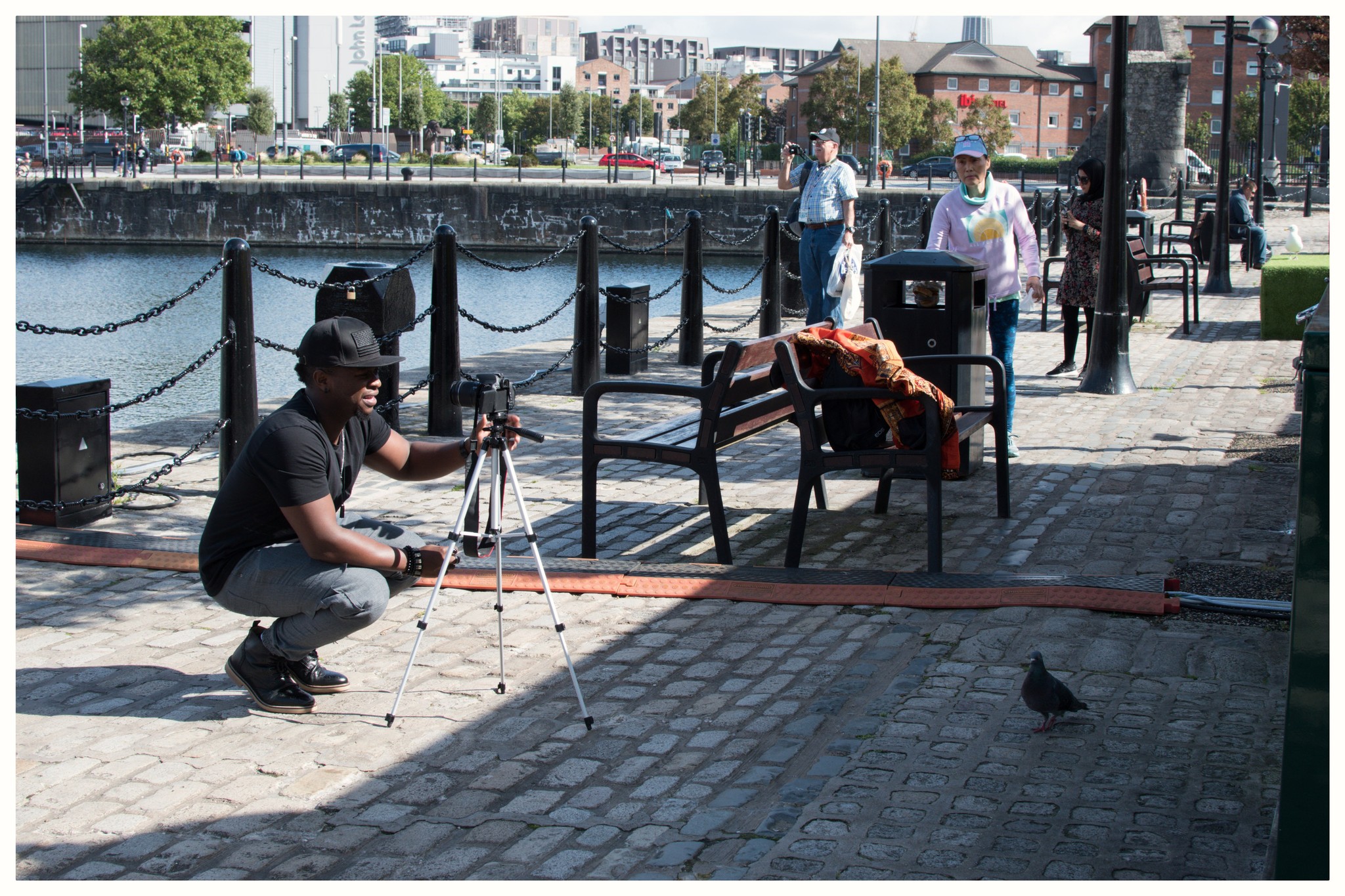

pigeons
left=1020, top=651, right=1088, bottom=733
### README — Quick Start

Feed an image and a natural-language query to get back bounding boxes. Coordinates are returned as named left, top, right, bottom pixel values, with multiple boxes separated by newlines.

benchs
left=774, top=318, right=1010, bottom=574
left=1126, top=237, right=1199, bottom=334
left=760, top=169, right=780, bottom=178
left=1158, top=212, right=1208, bottom=268
left=582, top=316, right=838, bottom=564
left=671, top=168, right=705, bottom=178
left=1041, top=257, right=1067, bottom=332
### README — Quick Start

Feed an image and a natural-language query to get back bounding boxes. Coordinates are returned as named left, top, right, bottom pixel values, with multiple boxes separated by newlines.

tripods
left=384, top=416, right=594, bottom=731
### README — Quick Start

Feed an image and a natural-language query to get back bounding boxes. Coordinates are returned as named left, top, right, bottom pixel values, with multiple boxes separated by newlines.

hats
left=951, top=134, right=987, bottom=160
left=295, top=316, right=406, bottom=367
left=810, top=128, right=840, bottom=147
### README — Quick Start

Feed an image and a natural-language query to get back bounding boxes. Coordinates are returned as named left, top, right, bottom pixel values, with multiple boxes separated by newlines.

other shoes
left=1253, top=263, right=1264, bottom=269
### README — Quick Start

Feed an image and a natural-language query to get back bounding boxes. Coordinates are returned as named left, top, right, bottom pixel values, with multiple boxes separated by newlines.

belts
left=805, top=219, right=845, bottom=230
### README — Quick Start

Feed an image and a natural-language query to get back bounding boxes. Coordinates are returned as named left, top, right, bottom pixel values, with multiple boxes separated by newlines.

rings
left=452, top=548, right=457, bottom=555
left=454, top=556, right=459, bottom=565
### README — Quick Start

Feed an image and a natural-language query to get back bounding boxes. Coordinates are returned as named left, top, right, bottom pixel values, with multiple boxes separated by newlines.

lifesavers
left=877, top=160, right=892, bottom=177
left=170, top=150, right=185, bottom=165
left=1140, top=177, right=1146, bottom=212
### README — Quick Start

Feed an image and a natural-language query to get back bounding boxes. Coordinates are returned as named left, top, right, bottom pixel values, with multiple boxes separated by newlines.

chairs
left=1211, top=212, right=1253, bottom=271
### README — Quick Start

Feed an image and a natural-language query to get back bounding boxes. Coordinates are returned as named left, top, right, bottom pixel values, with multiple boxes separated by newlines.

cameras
left=1057, top=201, right=1068, bottom=222
left=788, top=145, right=802, bottom=155
left=449, top=372, right=517, bottom=433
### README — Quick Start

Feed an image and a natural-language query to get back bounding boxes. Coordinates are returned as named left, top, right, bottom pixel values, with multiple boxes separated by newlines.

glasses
left=1075, top=174, right=1089, bottom=185
left=954, top=134, right=987, bottom=152
left=811, top=140, right=835, bottom=145
left=1251, top=191, right=1255, bottom=194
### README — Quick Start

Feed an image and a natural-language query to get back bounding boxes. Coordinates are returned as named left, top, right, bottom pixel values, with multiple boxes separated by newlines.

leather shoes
left=284, top=648, right=349, bottom=693
left=225, top=620, right=318, bottom=714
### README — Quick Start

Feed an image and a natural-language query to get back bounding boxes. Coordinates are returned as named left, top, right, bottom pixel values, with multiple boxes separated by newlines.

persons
left=16, top=152, right=33, bottom=177
left=192, top=143, right=200, bottom=156
left=217, top=143, right=224, bottom=162
left=199, top=316, right=523, bottom=715
left=229, top=144, right=247, bottom=178
left=1229, top=181, right=1267, bottom=270
left=778, top=128, right=859, bottom=330
left=135, top=140, right=149, bottom=173
left=921, top=134, right=1045, bottom=457
left=1046, top=159, right=1106, bottom=380
left=160, top=141, right=165, bottom=155
left=111, top=143, right=121, bottom=173
left=117, top=145, right=135, bottom=178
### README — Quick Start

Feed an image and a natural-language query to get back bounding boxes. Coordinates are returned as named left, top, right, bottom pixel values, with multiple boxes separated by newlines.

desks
left=1194, top=193, right=1216, bottom=221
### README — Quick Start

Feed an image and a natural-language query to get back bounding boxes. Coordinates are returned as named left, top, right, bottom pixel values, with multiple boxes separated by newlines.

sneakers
left=1045, top=360, right=1077, bottom=377
left=1007, top=431, right=1020, bottom=457
left=1077, top=363, right=1088, bottom=380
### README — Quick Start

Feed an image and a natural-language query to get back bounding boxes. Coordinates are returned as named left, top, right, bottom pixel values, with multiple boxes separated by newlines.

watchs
left=1083, top=224, right=1088, bottom=232
left=844, top=226, right=855, bottom=234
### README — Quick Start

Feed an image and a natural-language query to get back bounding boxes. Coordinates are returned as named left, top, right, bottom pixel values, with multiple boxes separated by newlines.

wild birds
left=1284, top=224, right=1302, bottom=260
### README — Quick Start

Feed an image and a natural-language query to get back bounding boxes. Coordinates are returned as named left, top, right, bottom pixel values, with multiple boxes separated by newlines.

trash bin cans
left=1123, top=209, right=1153, bottom=317
left=725, top=163, right=737, bottom=185
left=862, top=250, right=992, bottom=479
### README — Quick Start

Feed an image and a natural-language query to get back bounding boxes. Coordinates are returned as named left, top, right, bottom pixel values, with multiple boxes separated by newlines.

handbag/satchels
left=826, top=238, right=863, bottom=322
left=786, top=196, right=804, bottom=237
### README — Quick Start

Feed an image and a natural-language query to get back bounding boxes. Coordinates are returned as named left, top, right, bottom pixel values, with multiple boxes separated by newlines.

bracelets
left=459, top=437, right=470, bottom=457
left=401, top=546, right=423, bottom=580
